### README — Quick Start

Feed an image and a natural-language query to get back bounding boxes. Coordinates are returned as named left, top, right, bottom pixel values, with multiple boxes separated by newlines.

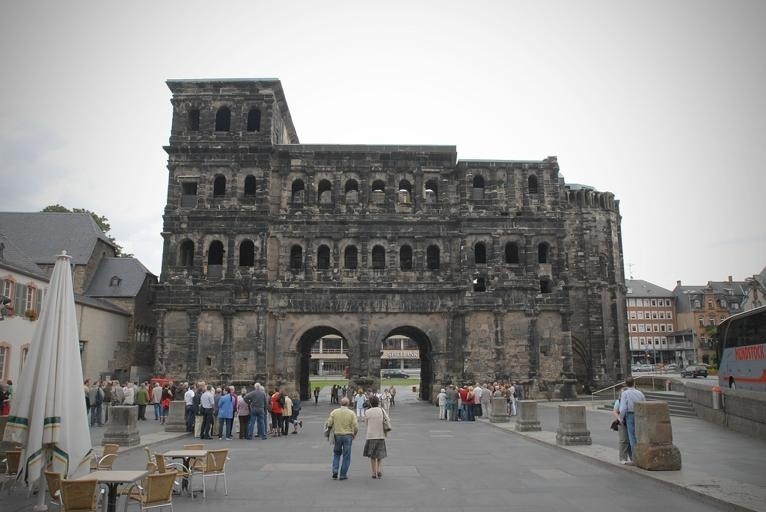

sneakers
left=332, top=473, right=381, bottom=479
left=619, top=461, right=628, bottom=465
left=298, top=421, right=302, bottom=428
left=292, top=431, right=297, bottom=434
left=198, top=429, right=288, bottom=440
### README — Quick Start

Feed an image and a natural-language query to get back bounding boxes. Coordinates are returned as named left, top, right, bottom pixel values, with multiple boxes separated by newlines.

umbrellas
left=2, top=248, right=92, bottom=501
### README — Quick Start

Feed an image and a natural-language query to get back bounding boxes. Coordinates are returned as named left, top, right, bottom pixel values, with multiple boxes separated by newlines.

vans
left=381, top=369, right=410, bottom=380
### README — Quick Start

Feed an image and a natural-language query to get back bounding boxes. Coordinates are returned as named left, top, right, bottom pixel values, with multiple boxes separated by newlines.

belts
left=628, top=412, right=634, bottom=414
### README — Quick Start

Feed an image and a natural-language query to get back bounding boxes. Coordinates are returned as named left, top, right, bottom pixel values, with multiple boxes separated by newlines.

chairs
left=0, top=440, right=231, bottom=512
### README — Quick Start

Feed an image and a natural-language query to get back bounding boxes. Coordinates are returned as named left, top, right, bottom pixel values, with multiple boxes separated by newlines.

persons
left=620, top=377, right=646, bottom=466
left=314, top=387, right=320, bottom=404
left=437, top=381, right=524, bottom=421
left=362, top=395, right=392, bottom=478
left=84, top=378, right=302, bottom=440
left=354, top=388, right=367, bottom=422
left=614, top=385, right=630, bottom=465
left=0, top=378, right=14, bottom=411
left=331, top=383, right=398, bottom=411
left=6, top=379, right=14, bottom=394
left=323, top=396, right=359, bottom=480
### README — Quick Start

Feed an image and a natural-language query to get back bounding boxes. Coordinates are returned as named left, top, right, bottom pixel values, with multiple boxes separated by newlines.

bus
left=714, top=303, right=765, bottom=396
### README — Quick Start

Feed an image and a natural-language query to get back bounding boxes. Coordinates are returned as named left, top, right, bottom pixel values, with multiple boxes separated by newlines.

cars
left=630, top=359, right=711, bottom=381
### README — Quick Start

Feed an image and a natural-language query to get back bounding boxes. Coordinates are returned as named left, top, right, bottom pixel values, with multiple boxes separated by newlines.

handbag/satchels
left=381, top=407, right=392, bottom=433
left=363, top=403, right=368, bottom=408
left=161, top=399, right=169, bottom=409
left=95, top=387, right=102, bottom=406
left=611, top=420, right=619, bottom=431
